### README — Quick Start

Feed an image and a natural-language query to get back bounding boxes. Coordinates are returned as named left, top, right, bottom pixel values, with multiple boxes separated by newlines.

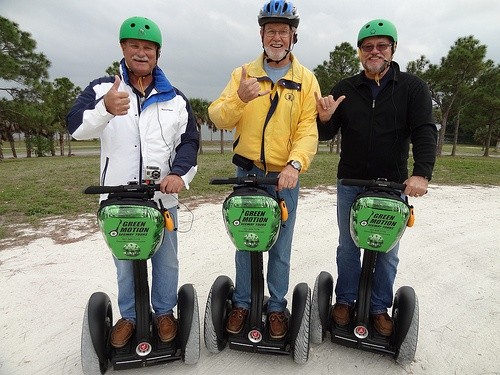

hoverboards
left=79, top=179, right=201, bottom=375
left=203, top=175, right=311, bottom=365
left=311, top=177, right=428, bottom=365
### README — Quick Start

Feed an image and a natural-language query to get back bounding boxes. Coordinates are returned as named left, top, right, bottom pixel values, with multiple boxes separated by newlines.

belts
left=375, top=177, right=393, bottom=187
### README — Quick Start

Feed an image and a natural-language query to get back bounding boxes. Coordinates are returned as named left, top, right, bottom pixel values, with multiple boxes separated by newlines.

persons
left=67, top=17, right=201, bottom=348
left=314, top=19, right=439, bottom=337
left=209, top=0, right=320, bottom=339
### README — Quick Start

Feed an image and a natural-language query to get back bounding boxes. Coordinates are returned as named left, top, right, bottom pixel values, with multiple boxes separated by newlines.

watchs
left=286, top=160, right=301, bottom=173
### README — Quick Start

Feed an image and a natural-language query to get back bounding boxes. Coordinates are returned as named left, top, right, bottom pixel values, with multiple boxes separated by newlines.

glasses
left=263, top=29, right=292, bottom=38
left=360, top=44, right=393, bottom=52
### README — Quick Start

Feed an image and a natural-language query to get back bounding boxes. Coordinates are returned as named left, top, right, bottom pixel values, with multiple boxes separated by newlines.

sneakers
left=110, top=318, right=134, bottom=348
left=269, top=310, right=287, bottom=338
left=226, top=307, right=249, bottom=333
left=158, top=313, right=176, bottom=342
left=373, top=312, right=392, bottom=336
left=334, top=302, right=353, bottom=325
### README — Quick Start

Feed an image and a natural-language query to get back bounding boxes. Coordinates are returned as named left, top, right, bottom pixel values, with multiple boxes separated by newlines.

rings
left=258, top=93, right=260, bottom=96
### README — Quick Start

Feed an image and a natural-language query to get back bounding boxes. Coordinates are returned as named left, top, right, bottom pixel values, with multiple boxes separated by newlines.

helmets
left=257, top=0, right=299, bottom=29
left=120, top=17, right=161, bottom=48
left=358, top=20, right=397, bottom=48
